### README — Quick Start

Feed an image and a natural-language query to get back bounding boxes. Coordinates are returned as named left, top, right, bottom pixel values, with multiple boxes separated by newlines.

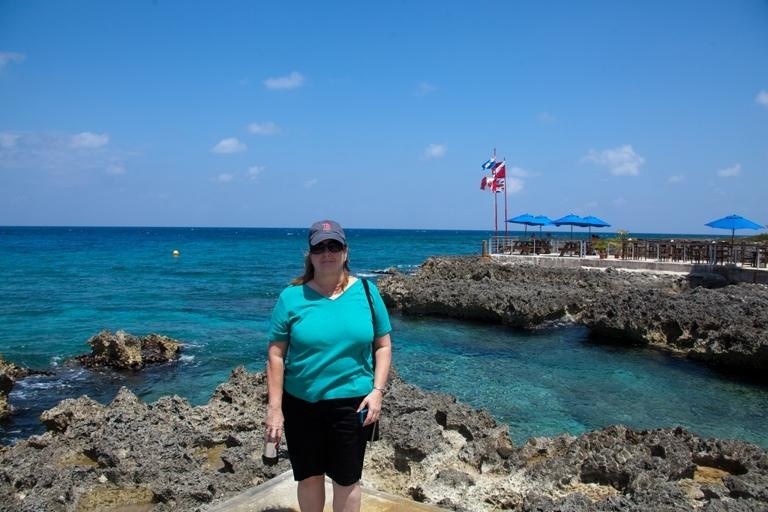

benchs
left=507, top=237, right=768, bottom=268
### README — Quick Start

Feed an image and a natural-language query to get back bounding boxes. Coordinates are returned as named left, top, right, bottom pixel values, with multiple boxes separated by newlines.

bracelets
left=372, top=386, right=385, bottom=394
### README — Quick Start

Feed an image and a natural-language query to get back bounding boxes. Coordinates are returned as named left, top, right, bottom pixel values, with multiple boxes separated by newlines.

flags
left=480, top=155, right=505, bottom=195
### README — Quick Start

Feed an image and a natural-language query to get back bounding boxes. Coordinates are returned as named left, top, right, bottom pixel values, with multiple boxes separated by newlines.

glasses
left=310, top=243, right=342, bottom=253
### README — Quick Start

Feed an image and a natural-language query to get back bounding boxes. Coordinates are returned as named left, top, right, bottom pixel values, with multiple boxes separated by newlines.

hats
left=310, top=220, right=346, bottom=246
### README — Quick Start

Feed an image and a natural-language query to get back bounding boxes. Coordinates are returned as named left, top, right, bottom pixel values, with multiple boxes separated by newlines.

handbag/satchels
left=361, top=277, right=381, bottom=450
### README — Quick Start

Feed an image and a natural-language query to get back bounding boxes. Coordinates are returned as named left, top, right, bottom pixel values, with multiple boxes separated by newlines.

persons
left=263, top=215, right=395, bottom=511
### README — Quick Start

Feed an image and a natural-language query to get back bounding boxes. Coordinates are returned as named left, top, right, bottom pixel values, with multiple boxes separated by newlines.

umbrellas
left=525, top=213, right=552, bottom=234
left=504, top=212, right=532, bottom=234
left=703, top=212, right=765, bottom=244
left=551, top=214, right=585, bottom=240
left=573, top=216, right=611, bottom=239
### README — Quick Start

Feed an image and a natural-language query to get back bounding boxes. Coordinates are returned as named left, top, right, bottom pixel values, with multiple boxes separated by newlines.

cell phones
left=360, top=409, right=368, bottom=424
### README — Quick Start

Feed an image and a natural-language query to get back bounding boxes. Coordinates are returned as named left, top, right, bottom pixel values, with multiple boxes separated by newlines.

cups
left=261, top=441, right=279, bottom=466
